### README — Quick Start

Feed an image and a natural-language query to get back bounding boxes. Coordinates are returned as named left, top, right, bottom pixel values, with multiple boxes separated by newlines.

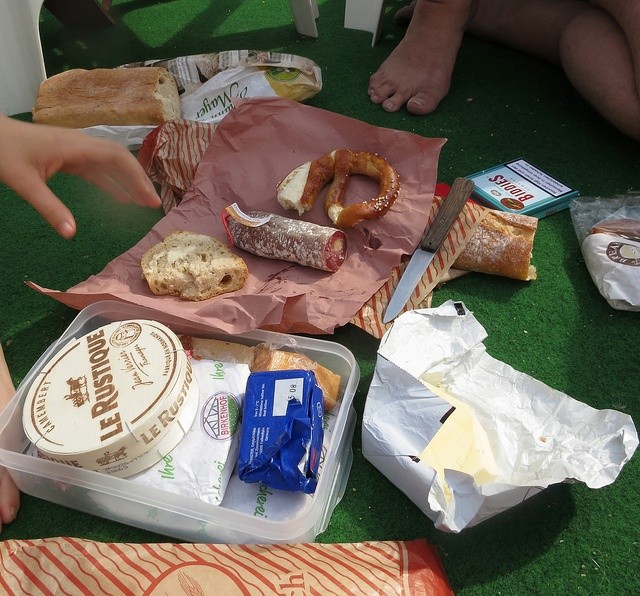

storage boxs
left=0, top=301, right=360, bottom=543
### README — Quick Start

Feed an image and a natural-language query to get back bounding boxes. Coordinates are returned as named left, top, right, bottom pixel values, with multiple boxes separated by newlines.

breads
left=34, top=66, right=181, bottom=129
left=274, top=147, right=402, bottom=224
left=568, top=189, right=640, bottom=315
left=452, top=208, right=538, bottom=282
left=139, top=231, right=248, bottom=302
left=254, top=348, right=339, bottom=409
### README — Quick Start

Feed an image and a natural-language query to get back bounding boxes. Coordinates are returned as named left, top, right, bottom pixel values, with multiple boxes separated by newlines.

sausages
left=222, top=203, right=347, bottom=272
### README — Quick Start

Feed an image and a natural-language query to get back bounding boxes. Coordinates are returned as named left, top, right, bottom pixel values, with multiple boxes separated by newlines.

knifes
left=379, top=177, right=476, bottom=324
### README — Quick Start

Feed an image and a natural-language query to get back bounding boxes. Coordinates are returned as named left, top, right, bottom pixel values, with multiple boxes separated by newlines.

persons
left=0, top=106, right=164, bottom=521
left=369, top=0, right=638, bottom=147
